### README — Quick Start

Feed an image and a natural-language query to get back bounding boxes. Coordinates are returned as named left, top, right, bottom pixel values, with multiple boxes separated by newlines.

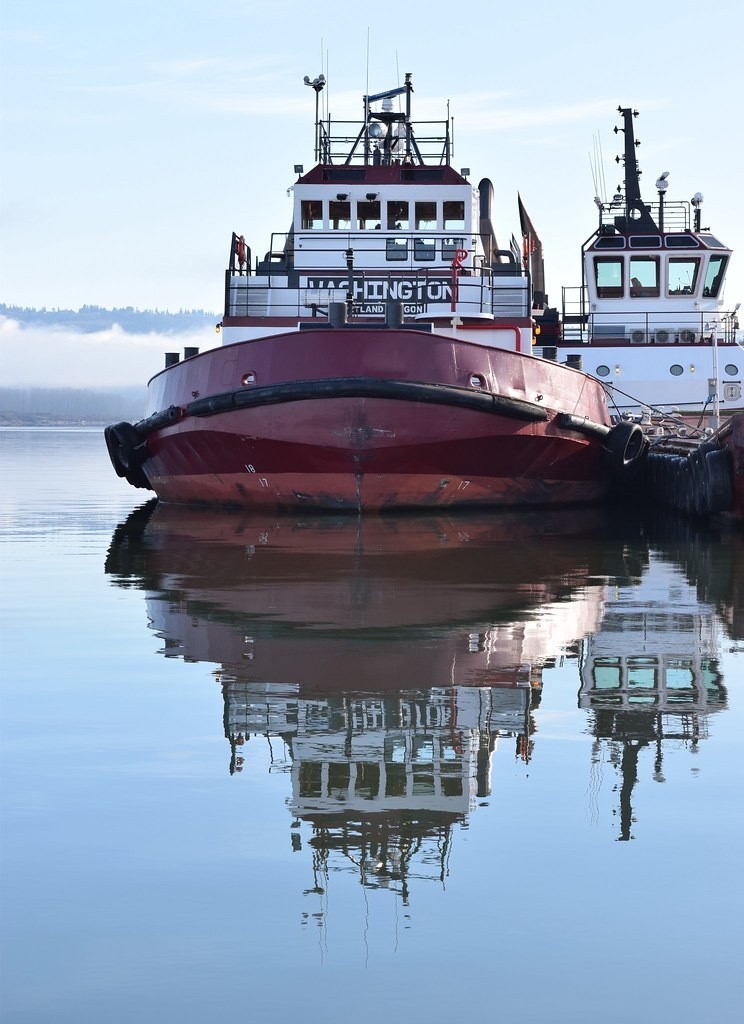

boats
left=558, top=105, right=744, bottom=526
left=105, top=496, right=652, bottom=964
left=104, top=28, right=658, bottom=511
left=577, top=527, right=744, bottom=842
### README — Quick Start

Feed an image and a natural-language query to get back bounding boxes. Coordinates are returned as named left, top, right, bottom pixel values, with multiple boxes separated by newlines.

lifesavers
left=605, top=421, right=645, bottom=468
left=104, top=422, right=155, bottom=491
left=455, top=248, right=468, bottom=261
left=638, top=441, right=736, bottom=519
left=238, top=235, right=246, bottom=256
